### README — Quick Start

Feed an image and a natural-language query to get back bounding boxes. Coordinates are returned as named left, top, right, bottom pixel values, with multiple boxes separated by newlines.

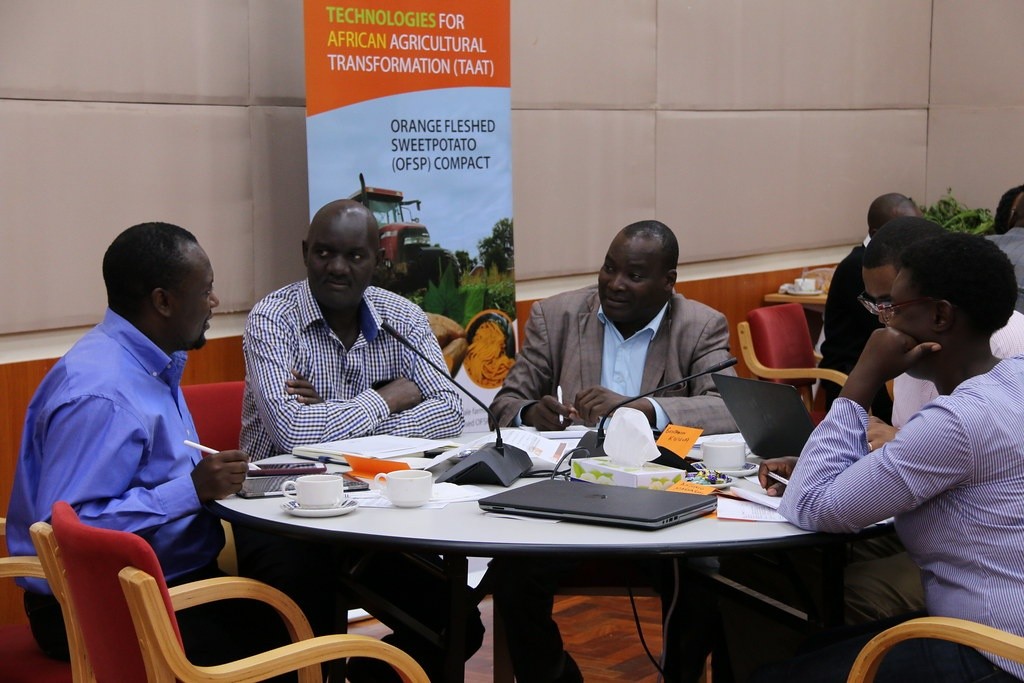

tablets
left=235, top=472, right=370, bottom=498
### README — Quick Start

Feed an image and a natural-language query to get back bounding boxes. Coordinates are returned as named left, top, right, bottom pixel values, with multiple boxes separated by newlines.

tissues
left=569, top=406, right=687, bottom=489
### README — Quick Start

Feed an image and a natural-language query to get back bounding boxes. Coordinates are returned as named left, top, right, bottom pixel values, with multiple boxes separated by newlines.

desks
left=765, top=293, right=828, bottom=311
left=228, top=430, right=914, bottom=683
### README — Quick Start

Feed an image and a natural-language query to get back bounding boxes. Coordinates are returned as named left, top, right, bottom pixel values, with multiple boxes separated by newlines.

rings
left=868, top=443, right=873, bottom=451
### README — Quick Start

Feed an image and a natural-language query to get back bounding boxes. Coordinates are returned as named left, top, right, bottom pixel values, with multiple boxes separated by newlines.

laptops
left=710, top=372, right=817, bottom=459
left=479, top=479, right=718, bottom=530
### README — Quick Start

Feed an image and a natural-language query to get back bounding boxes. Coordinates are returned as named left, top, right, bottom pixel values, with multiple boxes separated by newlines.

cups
left=702, top=440, right=745, bottom=470
left=281, top=475, right=344, bottom=509
left=795, top=279, right=815, bottom=292
left=374, top=470, right=432, bottom=507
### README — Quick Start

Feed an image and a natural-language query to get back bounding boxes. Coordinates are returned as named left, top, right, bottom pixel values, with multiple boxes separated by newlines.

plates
left=690, top=461, right=760, bottom=477
left=686, top=472, right=736, bottom=489
left=787, top=289, right=821, bottom=295
left=281, top=498, right=358, bottom=517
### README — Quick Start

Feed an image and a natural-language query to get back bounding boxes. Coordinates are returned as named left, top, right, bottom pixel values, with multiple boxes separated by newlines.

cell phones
left=245, top=461, right=327, bottom=477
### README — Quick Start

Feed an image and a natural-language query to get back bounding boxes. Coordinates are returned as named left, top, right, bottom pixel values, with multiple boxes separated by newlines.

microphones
left=380, top=321, right=533, bottom=486
left=569, top=357, right=739, bottom=473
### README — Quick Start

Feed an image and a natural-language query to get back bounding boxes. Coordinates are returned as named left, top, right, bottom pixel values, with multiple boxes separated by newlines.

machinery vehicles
left=346, top=173, right=464, bottom=297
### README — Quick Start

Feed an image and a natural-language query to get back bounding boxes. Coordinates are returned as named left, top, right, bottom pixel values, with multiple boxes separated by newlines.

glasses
left=878, top=297, right=940, bottom=324
left=857, top=291, right=895, bottom=319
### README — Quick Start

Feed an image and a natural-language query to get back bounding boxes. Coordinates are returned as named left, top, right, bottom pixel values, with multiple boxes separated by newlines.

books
left=291, top=434, right=426, bottom=466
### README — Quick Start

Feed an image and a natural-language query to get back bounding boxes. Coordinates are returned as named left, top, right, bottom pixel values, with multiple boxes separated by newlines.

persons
left=819, top=186, right=1024, bottom=423
left=4, top=199, right=1024, bottom=683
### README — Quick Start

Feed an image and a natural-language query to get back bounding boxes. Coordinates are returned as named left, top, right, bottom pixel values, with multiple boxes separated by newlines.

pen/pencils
left=183, top=439, right=263, bottom=470
left=557, top=385, right=564, bottom=424
left=766, top=471, right=789, bottom=485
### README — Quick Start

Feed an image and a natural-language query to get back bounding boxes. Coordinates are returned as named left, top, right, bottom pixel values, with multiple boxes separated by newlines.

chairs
left=847, top=616, right=1024, bottom=683
left=0, top=516, right=72, bottom=683
left=734, top=299, right=849, bottom=427
left=28, top=500, right=432, bottom=683
left=184, top=382, right=253, bottom=457
